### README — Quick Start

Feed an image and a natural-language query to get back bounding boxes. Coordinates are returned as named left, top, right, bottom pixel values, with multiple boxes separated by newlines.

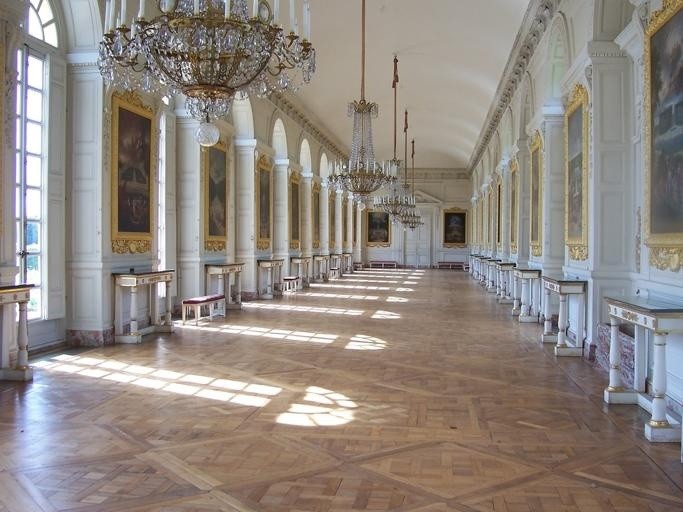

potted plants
left=443, top=206, right=468, bottom=248
left=644, top=0, right=683, bottom=272
left=366, top=209, right=391, bottom=247
left=497, top=82, right=589, bottom=260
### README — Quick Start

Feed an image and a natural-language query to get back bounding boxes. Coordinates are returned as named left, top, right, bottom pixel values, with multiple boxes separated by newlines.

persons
left=446, top=218, right=464, bottom=243
left=368, top=217, right=388, bottom=242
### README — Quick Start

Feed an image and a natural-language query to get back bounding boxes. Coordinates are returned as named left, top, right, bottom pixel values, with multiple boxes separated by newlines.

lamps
left=97, top=1, right=316, bottom=148
left=328, top=1, right=424, bottom=231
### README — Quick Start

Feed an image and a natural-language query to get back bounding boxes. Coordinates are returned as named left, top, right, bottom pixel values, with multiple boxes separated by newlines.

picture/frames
left=328, top=189, right=335, bottom=250
left=111, top=254, right=354, bottom=343
left=497, top=82, right=589, bottom=260
left=366, top=209, right=391, bottom=247
left=1, top=283, right=35, bottom=384
left=342, top=195, right=347, bottom=247
left=109, top=89, right=156, bottom=254
left=290, top=171, right=300, bottom=250
left=202, top=139, right=229, bottom=253
left=312, top=179, right=320, bottom=249
left=644, top=0, right=683, bottom=272
left=353, top=200, right=357, bottom=247
left=256, top=155, right=271, bottom=250
left=443, top=206, right=468, bottom=248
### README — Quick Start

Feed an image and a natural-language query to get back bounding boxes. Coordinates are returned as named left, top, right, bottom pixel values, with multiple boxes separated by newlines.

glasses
left=97, top=1, right=316, bottom=148
left=328, top=1, right=424, bottom=231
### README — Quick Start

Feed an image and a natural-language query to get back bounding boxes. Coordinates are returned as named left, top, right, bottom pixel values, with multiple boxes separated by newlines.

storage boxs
left=369, top=261, right=397, bottom=268
left=437, top=261, right=465, bottom=270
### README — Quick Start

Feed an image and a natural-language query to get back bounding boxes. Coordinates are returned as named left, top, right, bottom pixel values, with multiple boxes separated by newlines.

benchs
left=369, top=261, right=397, bottom=268
left=437, top=261, right=465, bottom=270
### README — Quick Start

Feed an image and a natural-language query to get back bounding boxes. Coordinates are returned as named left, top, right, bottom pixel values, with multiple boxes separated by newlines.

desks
left=111, top=254, right=354, bottom=343
left=1, top=283, right=35, bottom=384
left=603, top=296, right=682, bottom=441
left=542, top=273, right=588, bottom=357
left=469, top=254, right=517, bottom=304
left=512, top=266, right=542, bottom=323
